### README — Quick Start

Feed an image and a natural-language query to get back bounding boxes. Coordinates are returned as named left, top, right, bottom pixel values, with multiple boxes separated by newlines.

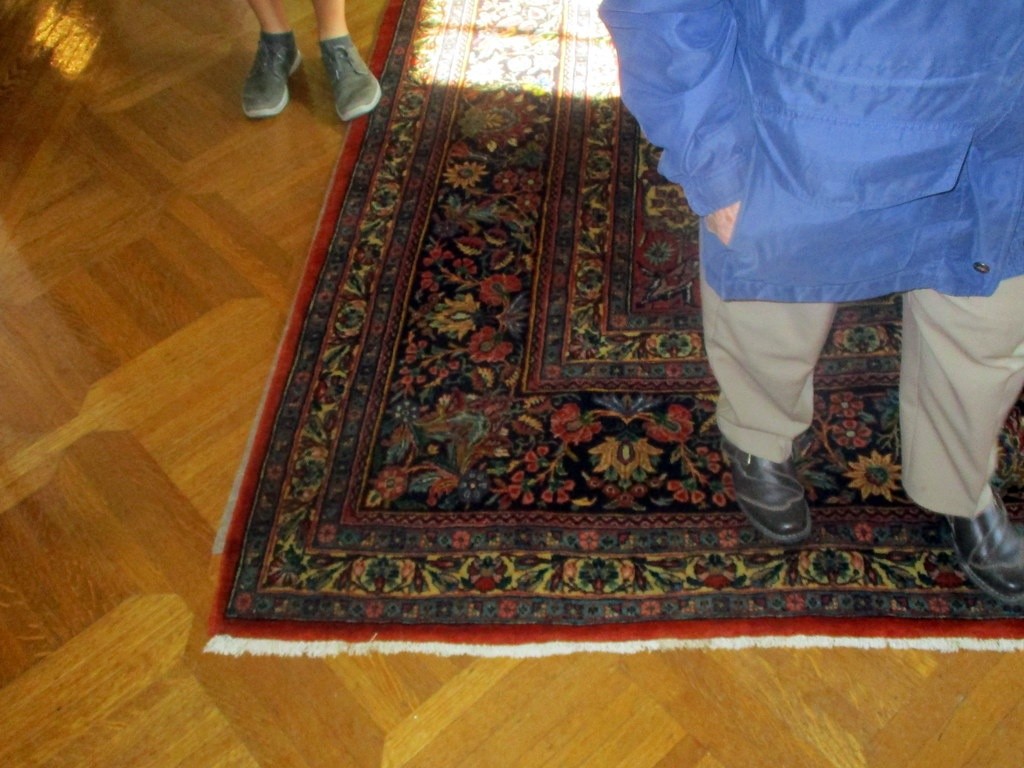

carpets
left=205, top=1, right=1022, bottom=660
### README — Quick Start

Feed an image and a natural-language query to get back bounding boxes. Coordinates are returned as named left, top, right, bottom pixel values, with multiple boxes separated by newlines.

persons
left=243, top=0, right=383, bottom=124
left=603, top=1, right=1024, bottom=612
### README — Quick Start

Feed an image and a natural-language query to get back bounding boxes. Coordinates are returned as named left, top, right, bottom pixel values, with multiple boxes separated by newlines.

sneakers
left=242, top=40, right=301, bottom=118
left=321, top=44, right=381, bottom=119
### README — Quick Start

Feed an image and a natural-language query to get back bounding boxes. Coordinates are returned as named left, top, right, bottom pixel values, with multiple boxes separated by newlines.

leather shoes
left=719, top=435, right=811, bottom=541
left=941, top=483, right=1024, bottom=602
